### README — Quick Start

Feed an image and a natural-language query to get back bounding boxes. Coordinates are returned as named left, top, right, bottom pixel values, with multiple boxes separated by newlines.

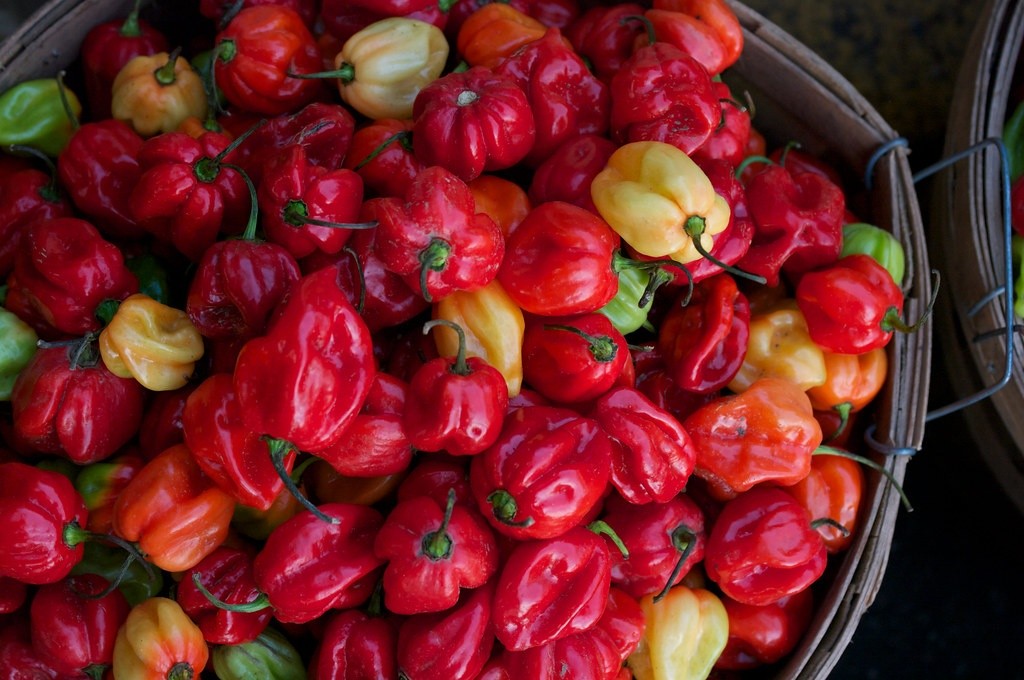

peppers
left=0, top=0, right=942, bottom=680
